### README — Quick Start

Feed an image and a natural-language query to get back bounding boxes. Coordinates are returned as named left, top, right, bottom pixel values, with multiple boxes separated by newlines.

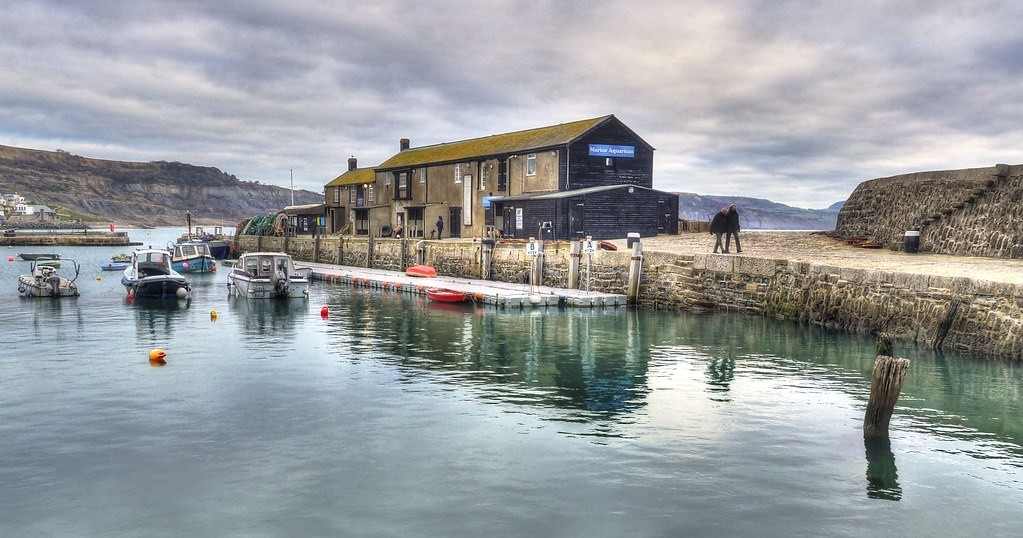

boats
left=164, top=237, right=216, bottom=274
left=100, top=254, right=133, bottom=271
left=16, top=252, right=62, bottom=261
left=405, top=264, right=438, bottom=278
left=122, top=245, right=193, bottom=304
left=290, top=261, right=316, bottom=298
left=16, top=257, right=82, bottom=297
left=424, top=287, right=477, bottom=304
left=226, top=252, right=292, bottom=302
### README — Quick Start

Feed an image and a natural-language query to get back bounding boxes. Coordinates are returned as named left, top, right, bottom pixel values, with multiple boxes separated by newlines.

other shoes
left=722, top=251, right=730, bottom=253
left=737, top=250, right=742, bottom=253
left=713, top=251, right=719, bottom=254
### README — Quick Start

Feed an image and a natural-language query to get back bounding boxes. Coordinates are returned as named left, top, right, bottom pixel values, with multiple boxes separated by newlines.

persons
left=710, top=208, right=727, bottom=254
left=436, top=216, right=443, bottom=240
left=310, top=223, right=316, bottom=238
left=724, top=204, right=742, bottom=253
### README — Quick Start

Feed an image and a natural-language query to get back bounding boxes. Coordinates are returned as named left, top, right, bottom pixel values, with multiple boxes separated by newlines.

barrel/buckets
left=627, top=232, right=639, bottom=248
left=904, top=230, right=919, bottom=253
